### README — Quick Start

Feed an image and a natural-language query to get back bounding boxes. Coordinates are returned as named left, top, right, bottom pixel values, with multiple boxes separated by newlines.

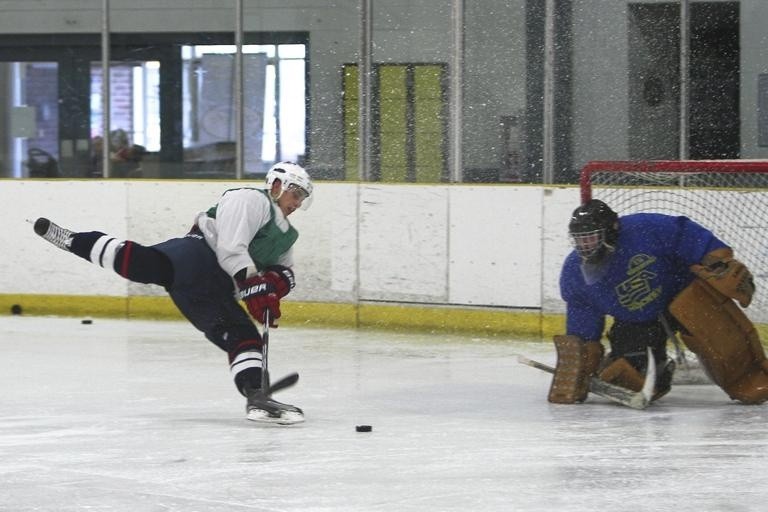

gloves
left=263, top=269, right=295, bottom=299
left=237, top=273, right=280, bottom=329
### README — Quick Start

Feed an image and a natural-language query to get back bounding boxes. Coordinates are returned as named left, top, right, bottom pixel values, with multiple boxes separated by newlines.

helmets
left=266, top=161, right=314, bottom=211
left=569, top=200, right=620, bottom=264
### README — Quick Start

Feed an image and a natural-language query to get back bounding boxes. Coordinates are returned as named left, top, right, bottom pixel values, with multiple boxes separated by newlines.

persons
left=88, top=137, right=105, bottom=178
left=106, top=126, right=135, bottom=180
left=30, top=159, right=316, bottom=425
left=542, top=197, right=767, bottom=406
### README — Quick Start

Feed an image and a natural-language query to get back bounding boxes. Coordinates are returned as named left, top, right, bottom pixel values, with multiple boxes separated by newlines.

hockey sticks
left=261, top=304, right=298, bottom=397
left=517, top=346, right=657, bottom=410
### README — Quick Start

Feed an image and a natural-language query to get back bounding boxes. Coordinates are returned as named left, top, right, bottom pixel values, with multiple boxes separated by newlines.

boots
left=240, top=377, right=303, bottom=417
left=34, top=218, right=77, bottom=252
